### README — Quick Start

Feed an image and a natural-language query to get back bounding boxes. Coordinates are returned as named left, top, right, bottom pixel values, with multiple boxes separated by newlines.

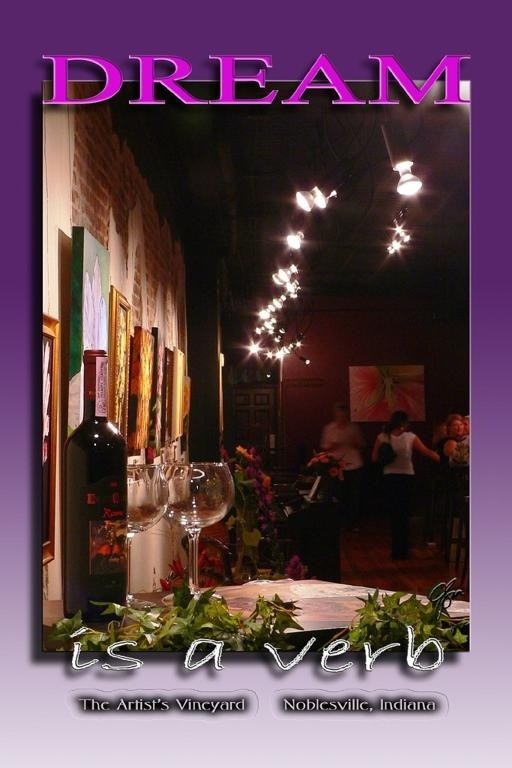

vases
left=320, top=480, right=336, bottom=496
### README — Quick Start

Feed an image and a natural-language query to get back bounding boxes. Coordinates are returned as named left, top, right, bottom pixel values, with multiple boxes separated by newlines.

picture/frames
left=42, top=313, right=61, bottom=565
left=255, top=393, right=269, bottom=405
left=235, top=394, right=249, bottom=406
left=108, top=286, right=133, bottom=433
left=234, top=410, right=249, bottom=423
left=255, top=409, right=268, bottom=421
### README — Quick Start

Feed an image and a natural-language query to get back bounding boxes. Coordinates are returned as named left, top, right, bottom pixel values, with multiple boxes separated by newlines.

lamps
left=250, top=183, right=337, bottom=377
left=380, top=124, right=422, bottom=196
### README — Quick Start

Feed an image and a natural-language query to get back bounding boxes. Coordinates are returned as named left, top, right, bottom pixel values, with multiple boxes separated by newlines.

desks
left=42, top=578, right=470, bottom=636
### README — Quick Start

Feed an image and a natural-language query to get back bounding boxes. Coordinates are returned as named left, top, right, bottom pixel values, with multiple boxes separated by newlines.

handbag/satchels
left=377, top=434, right=396, bottom=473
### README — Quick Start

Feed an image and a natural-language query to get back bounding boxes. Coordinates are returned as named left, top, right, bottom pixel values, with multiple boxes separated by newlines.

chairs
left=282, top=495, right=340, bottom=584
left=446, top=465, right=469, bottom=582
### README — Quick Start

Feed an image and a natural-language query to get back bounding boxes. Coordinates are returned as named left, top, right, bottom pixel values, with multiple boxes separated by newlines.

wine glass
left=124, top=460, right=170, bottom=607
left=151, top=459, right=237, bottom=603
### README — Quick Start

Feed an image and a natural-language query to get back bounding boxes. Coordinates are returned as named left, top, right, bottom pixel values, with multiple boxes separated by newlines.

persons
left=437, top=414, right=469, bottom=561
left=372, top=409, right=439, bottom=562
left=319, top=405, right=365, bottom=532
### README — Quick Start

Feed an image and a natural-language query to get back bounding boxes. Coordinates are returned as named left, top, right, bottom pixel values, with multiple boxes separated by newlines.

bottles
left=60, top=348, right=129, bottom=627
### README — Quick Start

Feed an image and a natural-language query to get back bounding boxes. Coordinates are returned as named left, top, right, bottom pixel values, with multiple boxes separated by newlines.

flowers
left=160, top=447, right=308, bottom=589
left=309, top=452, right=354, bottom=481
